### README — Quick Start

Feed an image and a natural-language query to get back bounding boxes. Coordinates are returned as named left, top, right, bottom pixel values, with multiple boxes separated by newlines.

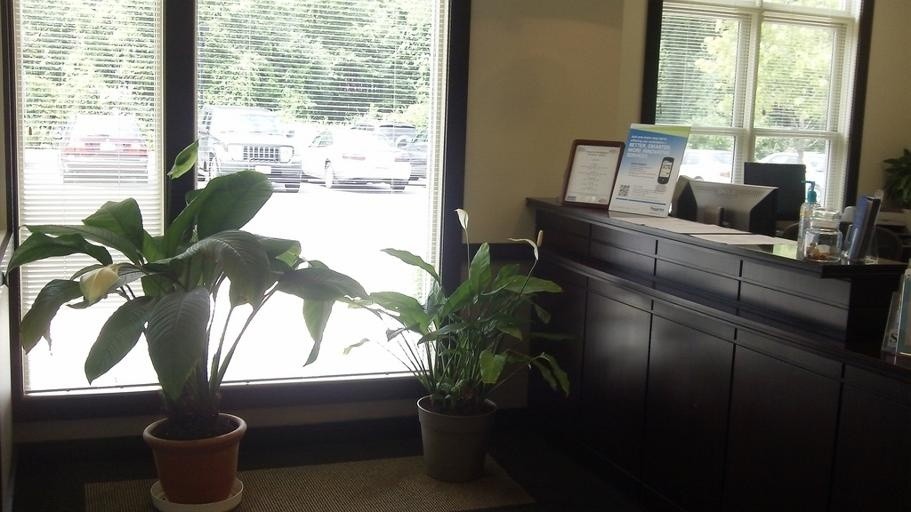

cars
left=53, top=114, right=149, bottom=186
left=302, top=119, right=428, bottom=191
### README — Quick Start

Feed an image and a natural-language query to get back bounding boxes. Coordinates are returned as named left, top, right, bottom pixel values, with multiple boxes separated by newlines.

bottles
left=803, top=208, right=843, bottom=263
left=848, top=195, right=882, bottom=263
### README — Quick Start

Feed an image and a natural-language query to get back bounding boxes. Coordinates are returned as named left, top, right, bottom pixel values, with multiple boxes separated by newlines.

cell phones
left=657, top=157, right=674, bottom=183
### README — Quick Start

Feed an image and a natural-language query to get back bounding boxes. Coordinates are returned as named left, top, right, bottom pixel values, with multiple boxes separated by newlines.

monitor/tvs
left=744, top=161, right=806, bottom=221
left=671, top=174, right=779, bottom=237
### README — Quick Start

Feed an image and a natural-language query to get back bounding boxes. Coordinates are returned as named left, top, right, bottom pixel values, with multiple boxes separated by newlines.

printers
left=840, top=204, right=911, bottom=263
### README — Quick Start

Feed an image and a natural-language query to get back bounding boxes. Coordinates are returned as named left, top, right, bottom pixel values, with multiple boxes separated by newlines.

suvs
left=198, top=103, right=302, bottom=193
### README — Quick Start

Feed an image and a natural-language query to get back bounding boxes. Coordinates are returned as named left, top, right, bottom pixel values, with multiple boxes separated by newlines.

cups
left=843, top=224, right=878, bottom=260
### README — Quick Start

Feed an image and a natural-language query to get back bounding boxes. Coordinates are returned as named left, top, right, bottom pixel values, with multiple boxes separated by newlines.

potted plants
left=344, top=208, right=569, bottom=482
left=4, top=141, right=373, bottom=505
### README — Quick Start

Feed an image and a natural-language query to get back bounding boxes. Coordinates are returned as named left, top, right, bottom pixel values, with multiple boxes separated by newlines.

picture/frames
left=559, top=139, right=627, bottom=209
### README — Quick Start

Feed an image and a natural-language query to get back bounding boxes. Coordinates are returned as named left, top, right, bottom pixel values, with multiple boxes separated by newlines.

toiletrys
left=797, top=179, right=821, bottom=258
